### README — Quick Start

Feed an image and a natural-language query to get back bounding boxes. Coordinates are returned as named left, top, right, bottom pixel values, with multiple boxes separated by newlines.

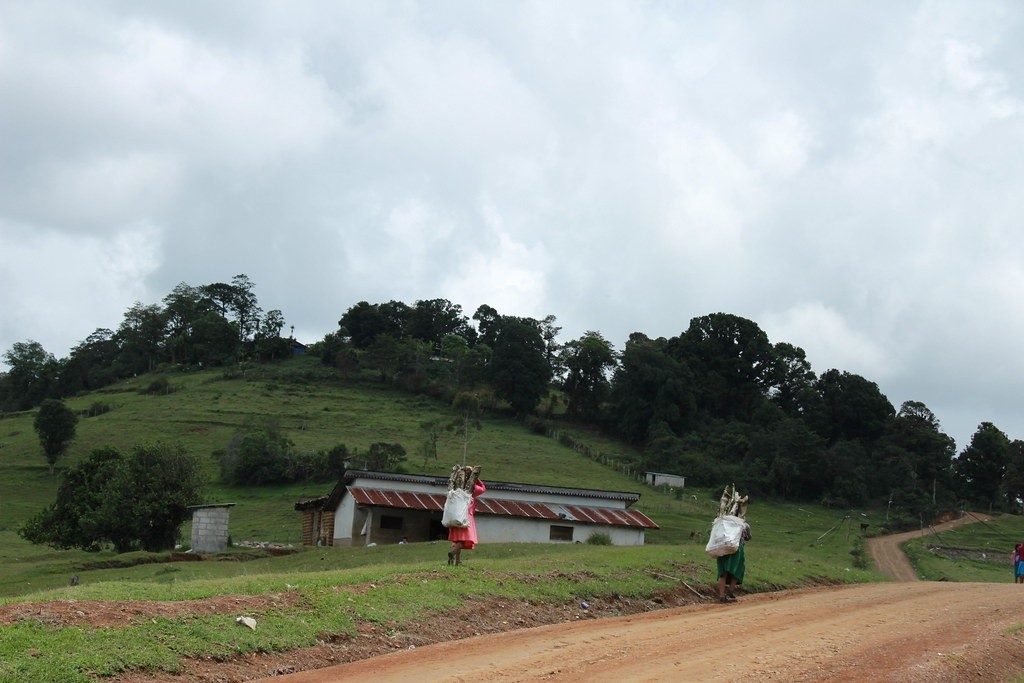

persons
left=1014, top=543, right=1024, bottom=583
left=717, top=506, right=752, bottom=603
left=447, top=466, right=486, bottom=566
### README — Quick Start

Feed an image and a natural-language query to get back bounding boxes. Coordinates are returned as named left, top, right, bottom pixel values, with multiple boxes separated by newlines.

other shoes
left=448, top=551, right=455, bottom=565
left=455, top=560, right=464, bottom=566
left=726, top=592, right=736, bottom=600
left=720, top=597, right=731, bottom=603
